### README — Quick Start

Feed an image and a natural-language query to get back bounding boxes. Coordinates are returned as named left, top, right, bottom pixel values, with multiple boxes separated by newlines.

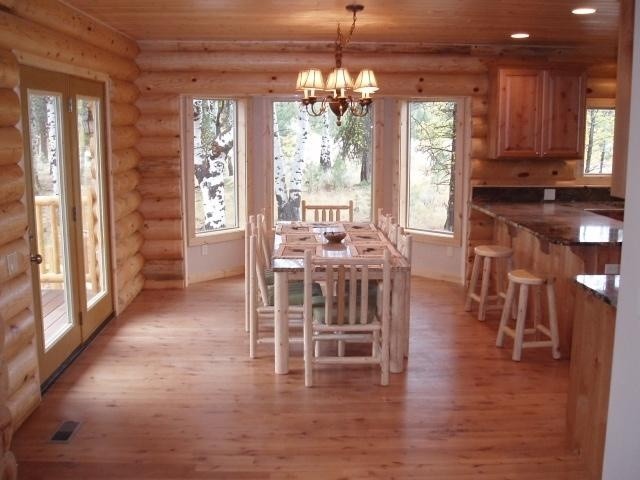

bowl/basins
left=324, top=231, right=347, bottom=243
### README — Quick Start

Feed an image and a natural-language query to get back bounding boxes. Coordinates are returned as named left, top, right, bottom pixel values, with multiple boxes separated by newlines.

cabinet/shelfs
left=488, top=63, right=588, bottom=161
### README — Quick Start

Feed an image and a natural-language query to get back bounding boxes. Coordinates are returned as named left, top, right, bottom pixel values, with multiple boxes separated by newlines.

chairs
left=245, top=200, right=412, bottom=389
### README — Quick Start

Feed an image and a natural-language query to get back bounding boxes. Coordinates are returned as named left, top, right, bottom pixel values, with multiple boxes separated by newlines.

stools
left=467, top=245, right=562, bottom=363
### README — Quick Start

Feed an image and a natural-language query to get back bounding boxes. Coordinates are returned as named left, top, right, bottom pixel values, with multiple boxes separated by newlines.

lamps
left=294, top=4, right=378, bottom=126
